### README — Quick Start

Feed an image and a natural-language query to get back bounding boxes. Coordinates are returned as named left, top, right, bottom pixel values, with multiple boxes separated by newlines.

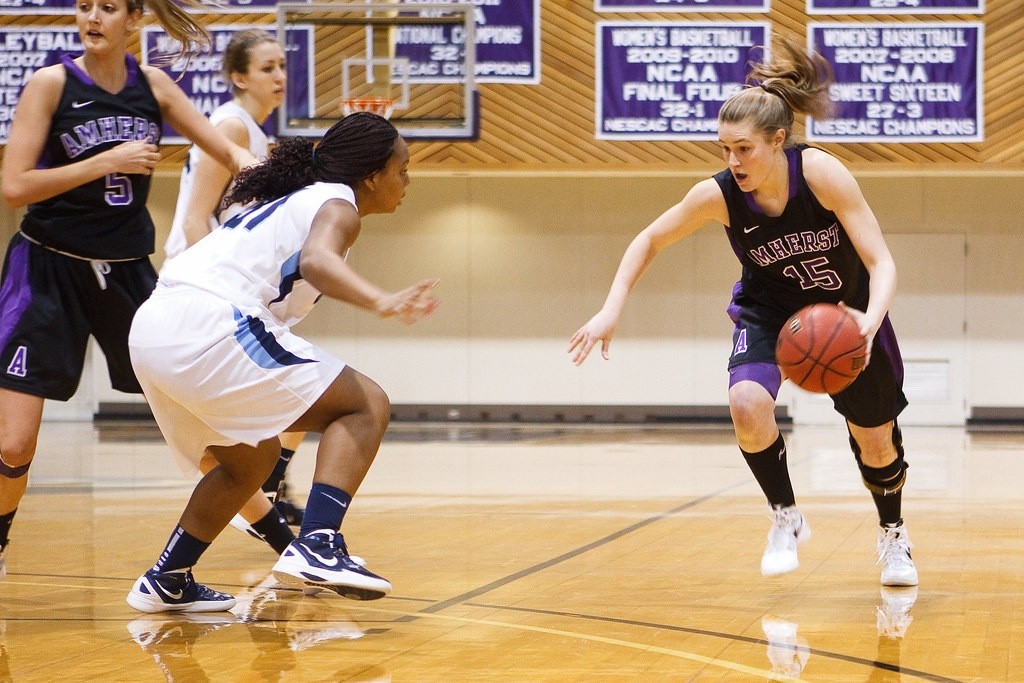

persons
left=0, top=595, right=391, bottom=683
left=762, top=586, right=918, bottom=683
left=0, top=0, right=440, bottom=614
left=569, top=31, right=919, bottom=586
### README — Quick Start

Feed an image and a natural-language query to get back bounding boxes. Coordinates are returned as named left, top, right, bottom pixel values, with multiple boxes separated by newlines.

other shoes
left=0, top=545, right=8, bottom=580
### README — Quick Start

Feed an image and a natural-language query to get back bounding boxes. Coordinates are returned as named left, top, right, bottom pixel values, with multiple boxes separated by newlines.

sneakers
left=762, top=620, right=810, bottom=678
left=126, top=567, right=237, bottom=613
left=873, top=518, right=919, bottom=585
left=876, top=586, right=919, bottom=639
left=229, top=492, right=305, bottom=542
left=127, top=611, right=237, bottom=653
left=226, top=573, right=323, bottom=624
left=272, top=529, right=392, bottom=601
left=761, top=503, right=811, bottom=576
left=288, top=610, right=364, bottom=652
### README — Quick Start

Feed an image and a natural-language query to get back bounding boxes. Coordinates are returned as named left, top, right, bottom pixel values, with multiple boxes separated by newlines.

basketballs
left=773, top=304, right=865, bottom=395
